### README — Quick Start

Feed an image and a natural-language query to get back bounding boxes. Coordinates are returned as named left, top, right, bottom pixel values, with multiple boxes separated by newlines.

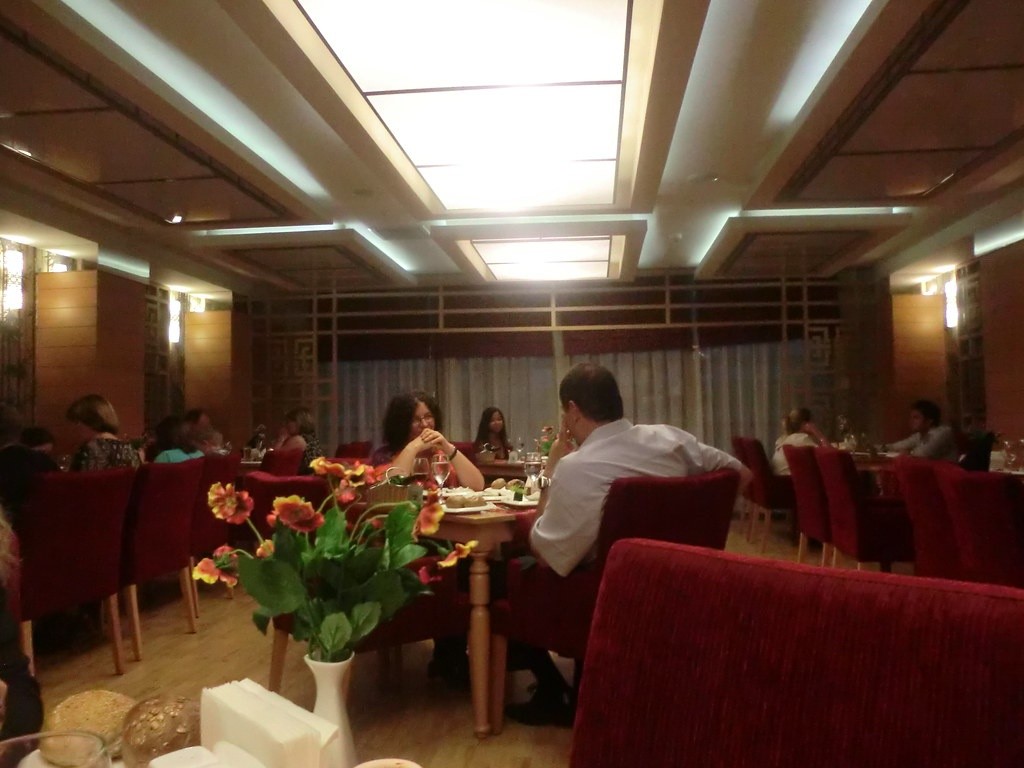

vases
left=304, top=648, right=357, bottom=768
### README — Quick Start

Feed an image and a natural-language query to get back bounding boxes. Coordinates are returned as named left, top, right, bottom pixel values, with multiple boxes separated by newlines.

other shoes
left=504, top=682, right=579, bottom=728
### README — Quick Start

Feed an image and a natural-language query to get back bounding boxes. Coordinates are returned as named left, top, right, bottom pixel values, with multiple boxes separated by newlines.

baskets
left=363, top=465, right=422, bottom=513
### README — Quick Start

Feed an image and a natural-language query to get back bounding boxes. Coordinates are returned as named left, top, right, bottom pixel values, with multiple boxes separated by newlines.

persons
left=769, top=408, right=832, bottom=552
left=0, top=395, right=325, bottom=524
left=0, top=516, right=44, bottom=768
left=505, top=362, right=754, bottom=728
left=467, top=407, right=513, bottom=465
left=365, top=389, right=485, bottom=680
left=875, top=400, right=959, bottom=462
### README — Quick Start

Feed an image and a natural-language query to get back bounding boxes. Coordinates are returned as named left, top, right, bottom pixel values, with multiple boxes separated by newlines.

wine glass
left=431, top=454, right=450, bottom=505
left=523, top=452, right=541, bottom=495
left=504, top=438, right=513, bottom=461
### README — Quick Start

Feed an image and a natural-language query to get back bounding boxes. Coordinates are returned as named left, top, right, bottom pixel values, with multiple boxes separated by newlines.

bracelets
left=909, top=449, right=914, bottom=456
left=449, top=446, right=458, bottom=460
left=817, top=439, right=826, bottom=443
left=278, top=439, right=284, bottom=442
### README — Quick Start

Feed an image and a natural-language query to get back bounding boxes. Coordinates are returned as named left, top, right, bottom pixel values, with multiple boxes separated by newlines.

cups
left=244, top=448, right=251, bottom=458
left=411, top=458, right=429, bottom=487
left=58, top=454, right=71, bottom=471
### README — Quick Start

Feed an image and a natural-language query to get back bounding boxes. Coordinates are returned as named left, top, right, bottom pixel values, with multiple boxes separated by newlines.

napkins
left=495, top=503, right=540, bottom=560
left=200, top=677, right=339, bottom=768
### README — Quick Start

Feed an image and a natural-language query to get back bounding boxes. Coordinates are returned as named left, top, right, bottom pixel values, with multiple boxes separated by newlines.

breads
left=491, top=477, right=522, bottom=490
left=445, top=494, right=486, bottom=508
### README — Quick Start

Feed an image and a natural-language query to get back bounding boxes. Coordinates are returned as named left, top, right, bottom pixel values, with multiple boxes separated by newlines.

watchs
left=538, top=475, right=552, bottom=489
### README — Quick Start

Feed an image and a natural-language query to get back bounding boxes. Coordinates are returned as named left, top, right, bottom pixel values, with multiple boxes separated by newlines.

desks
left=855, top=461, right=895, bottom=501
left=479, top=459, right=544, bottom=481
left=350, top=498, right=538, bottom=740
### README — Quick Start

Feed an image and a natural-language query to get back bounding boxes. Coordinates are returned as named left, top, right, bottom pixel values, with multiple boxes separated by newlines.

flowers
left=534, top=426, right=575, bottom=456
left=192, top=457, right=478, bottom=661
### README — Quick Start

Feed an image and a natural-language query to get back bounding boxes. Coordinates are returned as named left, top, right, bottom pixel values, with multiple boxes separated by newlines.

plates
left=850, top=452, right=887, bottom=456
left=423, top=488, right=538, bottom=513
left=241, top=458, right=255, bottom=461
left=989, top=468, right=1024, bottom=475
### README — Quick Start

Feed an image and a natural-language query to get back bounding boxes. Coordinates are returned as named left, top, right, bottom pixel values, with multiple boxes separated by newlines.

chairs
left=564, top=536, right=1024, bottom=768
left=0, top=436, right=480, bottom=679
left=491, top=466, right=741, bottom=733
left=731, top=434, right=1024, bottom=588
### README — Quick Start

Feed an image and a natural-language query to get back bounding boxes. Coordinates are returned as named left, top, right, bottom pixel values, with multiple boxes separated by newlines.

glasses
left=411, top=412, right=434, bottom=427
